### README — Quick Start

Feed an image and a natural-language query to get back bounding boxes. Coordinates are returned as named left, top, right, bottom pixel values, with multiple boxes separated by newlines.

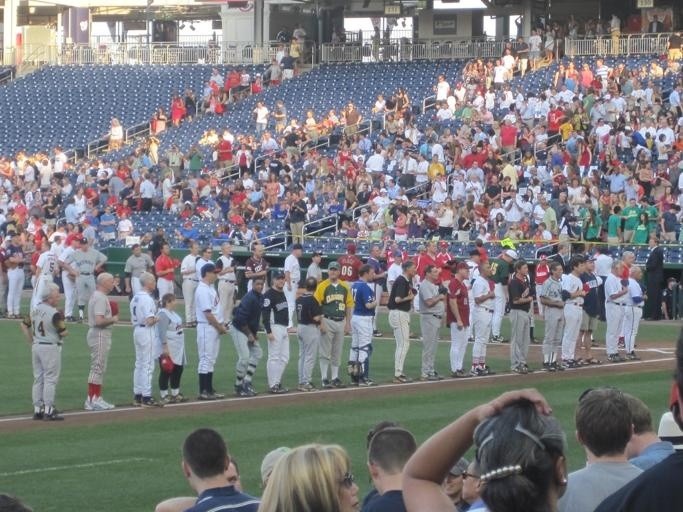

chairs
left=1, top=62, right=277, bottom=163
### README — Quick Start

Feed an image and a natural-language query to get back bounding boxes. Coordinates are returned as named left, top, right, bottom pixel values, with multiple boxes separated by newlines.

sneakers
left=210, top=389, right=225, bottom=400
left=551, top=360, right=565, bottom=370
left=83, top=399, right=108, bottom=411
left=331, top=378, right=347, bottom=388
left=561, top=359, right=582, bottom=368
left=132, top=397, right=142, bottom=407
left=350, top=377, right=359, bottom=385
left=607, top=354, right=626, bottom=362
left=420, top=372, right=445, bottom=381
left=359, top=377, right=378, bottom=387
left=298, top=382, right=320, bottom=392
left=142, top=398, right=165, bottom=408
left=34, top=412, right=44, bottom=420
left=41, top=412, right=64, bottom=422
left=7, top=314, right=23, bottom=319
left=452, top=369, right=474, bottom=377
left=169, top=393, right=192, bottom=403
left=470, top=366, right=496, bottom=375
left=269, top=385, right=288, bottom=393
left=626, top=352, right=641, bottom=360
left=322, top=379, right=336, bottom=388
left=245, top=383, right=259, bottom=395
left=513, top=364, right=535, bottom=374
left=392, top=374, right=413, bottom=384
left=540, top=363, right=556, bottom=372
left=158, top=394, right=174, bottom=405
left=198, top=391, right=217, bottom=399
left=97, top=396, right=115, bottom=410
left=234, top=387, right=257, bottom=396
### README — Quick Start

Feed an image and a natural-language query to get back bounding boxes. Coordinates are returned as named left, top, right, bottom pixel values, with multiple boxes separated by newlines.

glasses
left=340, top=476, right=354, bottom=490
left=462, top=470, right=480, bottom=481
left=578, top=384, right=618, bottom=404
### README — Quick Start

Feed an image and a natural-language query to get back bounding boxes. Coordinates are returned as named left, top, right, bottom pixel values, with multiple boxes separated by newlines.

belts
left=614, top=302, right=626, bottom=306
left=324, top=315, right=345, bottom=322
left=573, top=302, right=583, bottom=307
left=486, top=308, right=494, bottom=313
left=433, top=315, right=442, bottom=319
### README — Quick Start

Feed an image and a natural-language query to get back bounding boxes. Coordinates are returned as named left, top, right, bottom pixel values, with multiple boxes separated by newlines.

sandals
left=576, top=357, right=590, bottom=365
left=586, top=357, right=603, bottom=365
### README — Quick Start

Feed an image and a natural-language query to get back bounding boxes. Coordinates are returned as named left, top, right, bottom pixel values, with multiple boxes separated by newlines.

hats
left=109, top=300, right=119, bottom=316
left=160, top=355, right=175, bottom=374
left=11, top=232, right=21, bottom=238
left=584, top=255, right=597, bottom=262
left=72, top=233, right=85, bottom=241
left=394, top=252, right=402, bottom=257
left=202, top=264, right=221, bottom=273
left=668, top=376, right=680, bottom=409
left=344, top=244, right=356, bottom=252
left=505, top=249, right=519, bottom=260
left=272, top=270, right=286, bottom=278
left=657, top=411, right=683, bottom=450
left=80, top=237, right=88, bottom=245
left=455, top=262, right=473, bottom=270
left=450, top=458, right=469, bottom=476
left=328, top=261, right=341, bottom=271
left=312, top=250, right=323, bottom=256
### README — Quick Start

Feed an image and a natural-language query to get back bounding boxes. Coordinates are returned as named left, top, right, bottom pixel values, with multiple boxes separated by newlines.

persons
left=0, top=13, right=683, bottom=417
left=157, top=338, right=683, bottom=511
